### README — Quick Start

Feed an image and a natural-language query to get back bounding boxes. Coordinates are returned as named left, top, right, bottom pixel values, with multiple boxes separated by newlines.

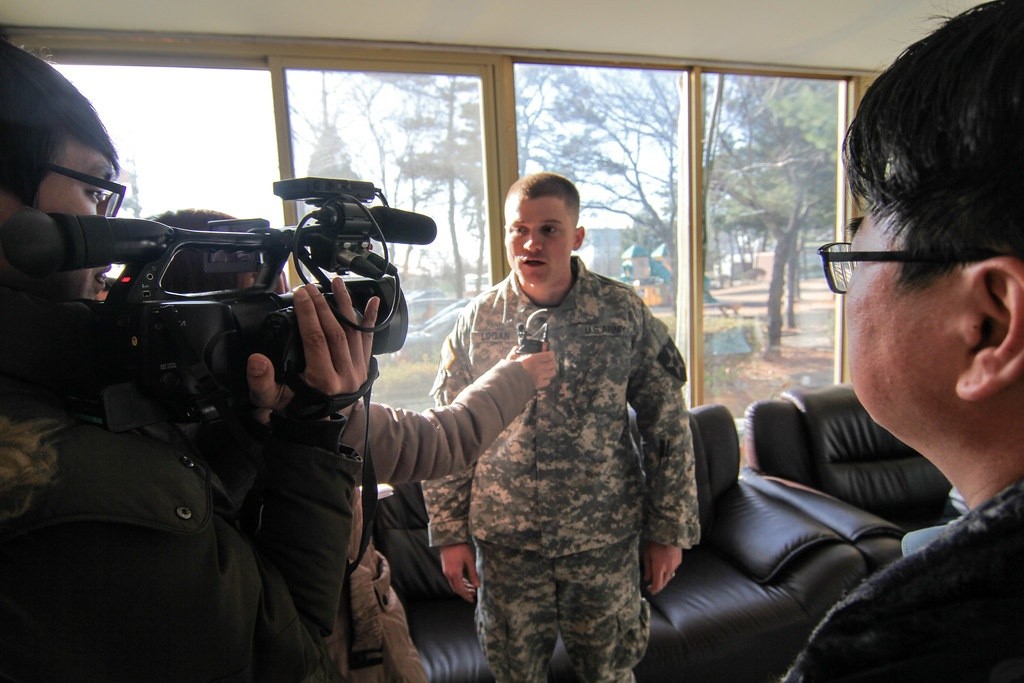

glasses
left=817, top=241, right=999, bottom=294
left=39, top=161, right=126, bottom=217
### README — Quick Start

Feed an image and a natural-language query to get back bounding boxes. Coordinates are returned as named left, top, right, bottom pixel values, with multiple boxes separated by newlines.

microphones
left=313, top=206, right=437, bottom=245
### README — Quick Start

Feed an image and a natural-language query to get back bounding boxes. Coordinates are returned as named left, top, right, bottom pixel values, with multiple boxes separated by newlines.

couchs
left=375, top=403, right=867, bottom=682
left=741, top=385, right=961, bottom=576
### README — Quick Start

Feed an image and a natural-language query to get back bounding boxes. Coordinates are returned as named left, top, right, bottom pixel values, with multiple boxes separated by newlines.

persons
left=1, top=36, right=381, bottom=683
left=779, top=0, right=1024, bottom=683
left=422, top=173, right=704, bottom=683
left=136, top=207, right=559, bottom=683
